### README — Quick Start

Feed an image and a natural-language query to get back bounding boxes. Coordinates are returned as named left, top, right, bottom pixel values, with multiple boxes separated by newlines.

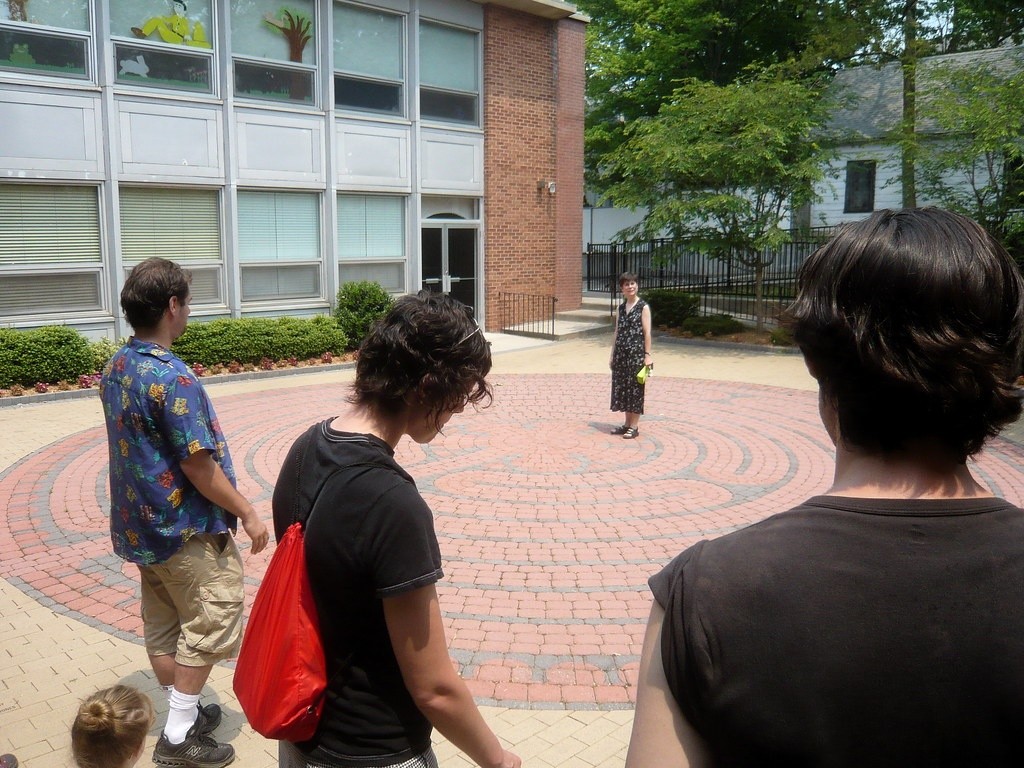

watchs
left=645, top=352, right=651, bottom=356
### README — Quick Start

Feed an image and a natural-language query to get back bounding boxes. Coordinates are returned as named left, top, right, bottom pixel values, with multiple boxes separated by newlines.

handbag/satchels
left=636, top=366, right=650, bottom=384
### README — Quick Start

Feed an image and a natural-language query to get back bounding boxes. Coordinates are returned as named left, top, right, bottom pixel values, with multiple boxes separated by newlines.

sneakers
left=153, top=730, right=235, bottom=768
left=194, top=700, right=222, bottom=733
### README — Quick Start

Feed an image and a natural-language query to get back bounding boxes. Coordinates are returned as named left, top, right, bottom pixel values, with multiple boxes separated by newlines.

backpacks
left=233, top=426, right=417, bottom=741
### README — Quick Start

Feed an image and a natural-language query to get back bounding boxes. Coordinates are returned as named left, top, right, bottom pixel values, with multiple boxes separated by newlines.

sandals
left=611, top=425, right=630, bottom=434
left=623, top=426, right=639, bottom=439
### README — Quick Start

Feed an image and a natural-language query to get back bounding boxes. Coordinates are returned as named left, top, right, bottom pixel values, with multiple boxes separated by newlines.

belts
left=296, top=739, right=431, bottom=766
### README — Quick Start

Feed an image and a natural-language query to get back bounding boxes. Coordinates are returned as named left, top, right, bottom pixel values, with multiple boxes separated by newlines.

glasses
left=449, top=318, right=483, bottom=355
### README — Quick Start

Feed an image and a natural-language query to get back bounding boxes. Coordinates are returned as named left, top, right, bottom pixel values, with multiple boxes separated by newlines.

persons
left=99, top=256, right=270, bottom=768
left=626, top=207, right=1024, bottom=768
left=72, top=684, right=156, bottom=768
left=271, top=292, right=522, bottom=768
left=608, top=272, right=654, bottom=439
